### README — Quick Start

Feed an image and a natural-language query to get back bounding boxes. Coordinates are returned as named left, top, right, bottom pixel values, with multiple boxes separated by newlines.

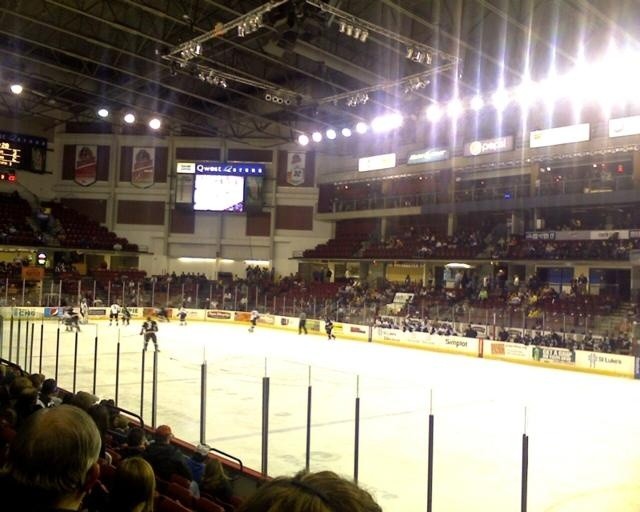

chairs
left=1, top=421, right=240, bottom=512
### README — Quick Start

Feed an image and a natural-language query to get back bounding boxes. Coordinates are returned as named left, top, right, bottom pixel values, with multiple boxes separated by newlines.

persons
left=139, top=316, right=160, bottom=352
left=2, top=359, right=386, bottom=509
left=109, top=302, right=121, bottom=326
left=121, top=304, right=131, bottom=325
left=298, top=308, right=308, bottom=335
left=65, top=309, right=78, bottom=330
left=157, top=307, right=170, bottom=322
left=80, top=297, right=88, bottom=324
left=322, top=141, right=637, bottom=359
left=176, top=306, right=188, bottom=326
left=2, top=188, right=322, bottom=320
left=152, top=309, right=166, bottom=322
left=63, top=306, right=81, bottom=331
left=248, top=307, right=261, bottom=332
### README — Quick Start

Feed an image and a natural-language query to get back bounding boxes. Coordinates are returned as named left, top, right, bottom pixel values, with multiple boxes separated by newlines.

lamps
left=405, top=46, right=432, bottom=65
left=237, top=13, right=264, bottom=36
left=403, top=73, right=432, bottom=97
left=194, top=72, right=227, bottom=88
left=180, top=43, right=201, bottom=61
left=345, top=91, right=369, bottom=107
left=337, top=18, right=369, bottom=42
left=262, top=89, right=291, bottom=107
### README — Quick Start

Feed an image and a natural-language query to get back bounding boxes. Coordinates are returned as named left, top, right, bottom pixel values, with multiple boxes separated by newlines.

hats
left=27, top=374, right=45, bottom=385
left=195, top=444, right=209, bottom=456
left=156, top=425, right=171, bottom=437
left=40, top=379, right=60, bottom=394
left=77, top=392, right=99, bottom=401
